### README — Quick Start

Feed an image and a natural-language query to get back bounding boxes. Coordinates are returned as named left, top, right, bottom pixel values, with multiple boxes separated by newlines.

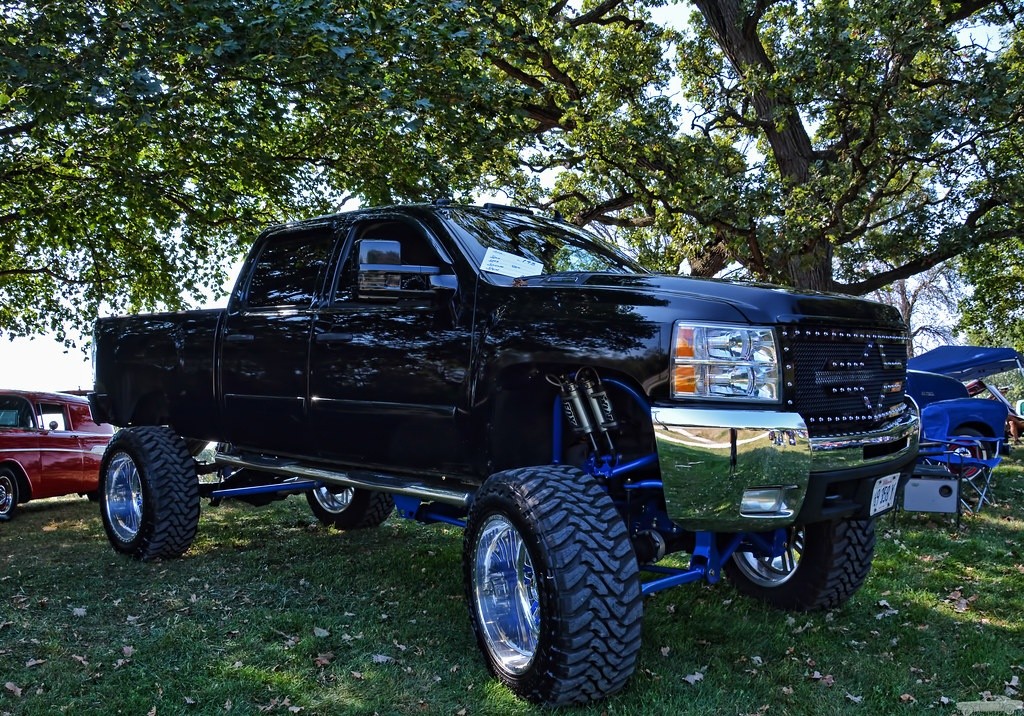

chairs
left=914, top=405, right=1005, bottom=514
left=890, top=443, right=964, bottom=527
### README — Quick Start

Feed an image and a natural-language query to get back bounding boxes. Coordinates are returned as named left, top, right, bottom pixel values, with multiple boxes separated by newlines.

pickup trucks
left=89, top=202, right=923, bottom=705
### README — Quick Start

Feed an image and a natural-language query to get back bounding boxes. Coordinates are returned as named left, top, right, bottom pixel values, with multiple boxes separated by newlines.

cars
left=904, top=346, right=1024, bottom=481
left=0, top=389, right=116, bottom=522
